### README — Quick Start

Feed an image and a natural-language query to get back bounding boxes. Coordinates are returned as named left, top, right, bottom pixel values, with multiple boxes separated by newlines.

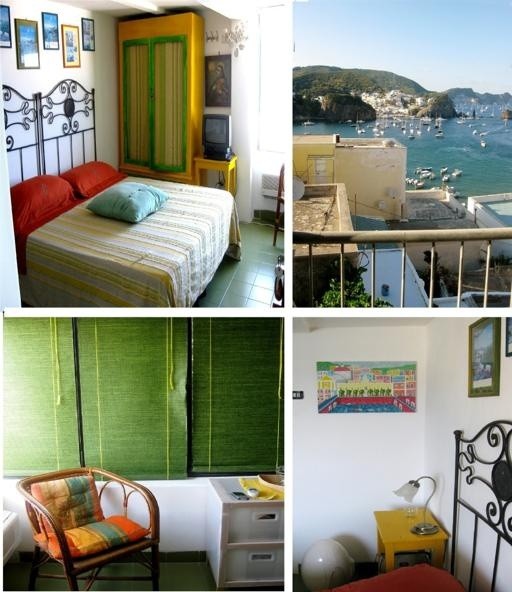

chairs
left=273, top=165, right=284, bottom=244
left=17, top=466, right=160, bottom=591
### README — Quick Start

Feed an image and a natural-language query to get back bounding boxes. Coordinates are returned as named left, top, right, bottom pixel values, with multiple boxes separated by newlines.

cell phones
left=231, top=492, right=249, bottom=500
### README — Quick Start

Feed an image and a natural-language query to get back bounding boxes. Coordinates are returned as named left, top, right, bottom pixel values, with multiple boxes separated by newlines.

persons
left=208, top=61, right=229, bottom=105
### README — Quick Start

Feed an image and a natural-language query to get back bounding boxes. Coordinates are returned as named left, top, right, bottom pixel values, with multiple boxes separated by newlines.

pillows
left=28, top=475, right=103, bottom=531
left=60, top=161, right=116, bottom=198
left=86, top=182, right=175, bottom=223
left=46, top=515, right=146, bottom=558
left=10, top=174, right=73, bottom=222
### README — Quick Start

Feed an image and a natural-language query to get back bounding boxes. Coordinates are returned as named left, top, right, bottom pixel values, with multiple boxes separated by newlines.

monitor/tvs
left=202, top=114, right=235, bottom=162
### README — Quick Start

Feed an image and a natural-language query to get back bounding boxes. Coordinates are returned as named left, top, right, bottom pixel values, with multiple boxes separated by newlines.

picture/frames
left=62, top=24, right=81, bottom=68
left=467, top=317, right=501, bottom=397
left=505, top=317, right=512, bottom=357
left=205, top=54, right=232, bottom=107
left=0, top=4, right=12, bottom=48
left=42, top=12, right=59, bottom=50
left=81, top=18, right=95, bottom=51
left=15, top=18, right=40, bottom=69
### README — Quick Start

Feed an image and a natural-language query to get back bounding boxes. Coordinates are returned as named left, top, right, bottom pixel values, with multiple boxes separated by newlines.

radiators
left=261, top=170, right=285, bottom=200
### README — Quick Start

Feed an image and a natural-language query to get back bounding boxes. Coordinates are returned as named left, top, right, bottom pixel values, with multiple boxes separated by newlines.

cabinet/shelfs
left=117, top=12, right=203, bottom=183
left=205, top=476, right=284, bottom=590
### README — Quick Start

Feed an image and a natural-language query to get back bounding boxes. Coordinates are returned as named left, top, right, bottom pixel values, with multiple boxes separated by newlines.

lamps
left=393, top=476, right=439, bottom=536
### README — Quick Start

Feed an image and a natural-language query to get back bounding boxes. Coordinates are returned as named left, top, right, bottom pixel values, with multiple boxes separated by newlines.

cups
left=275, top=465, right=284, bottom=486
left=405, top=505, right=416, bottom=519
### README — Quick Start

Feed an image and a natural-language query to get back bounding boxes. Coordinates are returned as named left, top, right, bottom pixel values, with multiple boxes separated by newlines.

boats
left=303, top=121, right=316, bottom=126
left=406, top=163, right=463, bottom=198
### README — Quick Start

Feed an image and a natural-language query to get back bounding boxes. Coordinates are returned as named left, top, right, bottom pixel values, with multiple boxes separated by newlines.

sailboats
left=341, top=99, right=509, bottom=149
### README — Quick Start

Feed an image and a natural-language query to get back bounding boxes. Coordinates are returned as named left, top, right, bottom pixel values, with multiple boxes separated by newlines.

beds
left=194, top=155, right=237, bottom=192
left=320, top=420, right=512, bottom=591
left=2, top=78, right=242, bottom=307
